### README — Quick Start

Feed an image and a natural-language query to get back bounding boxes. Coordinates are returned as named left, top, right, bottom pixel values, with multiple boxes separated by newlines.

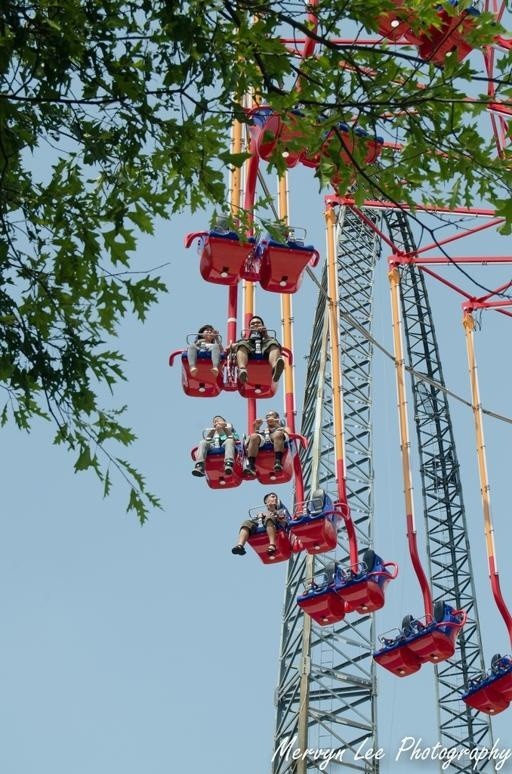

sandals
left=232, top=544, right=246, bottom=555
left=266, top=543, right=277, bottom=553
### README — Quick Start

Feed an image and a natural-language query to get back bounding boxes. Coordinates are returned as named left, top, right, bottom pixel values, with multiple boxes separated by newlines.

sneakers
left=239, top=368, right=247, bottom=383
left=271, top=358, right=284, bottom=381
left=244, top=464, right=256, bottom=475
left=273, top=460, right=283, bottom=472
left=211, top=367, right=219, bottom=377
left=190, top=367, right=199, bottom=376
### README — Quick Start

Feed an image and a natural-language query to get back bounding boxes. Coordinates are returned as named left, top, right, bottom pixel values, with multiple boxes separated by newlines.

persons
left=244, top=411, right=292, bottom=477
left=186, top=326, right=222, bottom=378
left=234, top=316, right=285, bottom=383
left=192, top=416, right=239, bottom=477
left=232, top=493, right=287, bottom=554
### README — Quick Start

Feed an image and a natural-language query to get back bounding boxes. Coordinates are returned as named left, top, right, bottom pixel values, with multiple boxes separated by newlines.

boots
left=224, top=462, right=233, bottom=474
left=192, top=462, right=206, bottom=476
